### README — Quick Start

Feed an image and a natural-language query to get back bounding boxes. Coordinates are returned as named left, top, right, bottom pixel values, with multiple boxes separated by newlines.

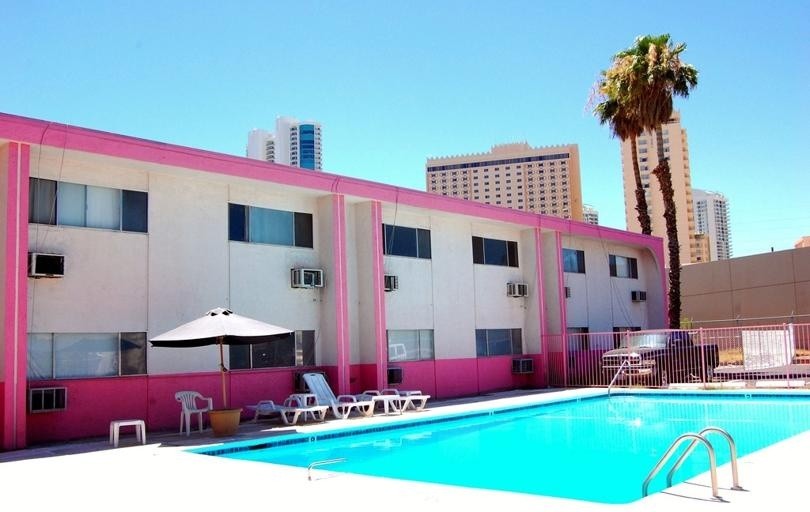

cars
left=388, top=342, right=407, bottom=363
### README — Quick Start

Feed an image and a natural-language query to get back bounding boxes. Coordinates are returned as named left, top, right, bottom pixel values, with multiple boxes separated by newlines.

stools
left=109, top=416, right=146, bottom=449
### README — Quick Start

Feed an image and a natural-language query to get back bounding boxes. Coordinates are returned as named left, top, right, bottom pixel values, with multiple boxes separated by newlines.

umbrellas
left=148, top=305, right=296, bottom=411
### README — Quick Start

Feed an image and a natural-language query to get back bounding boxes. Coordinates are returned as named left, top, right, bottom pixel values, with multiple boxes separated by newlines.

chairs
left=246, top=371, right=431, bottom=429
left=173, top=390, right=214, bottom=437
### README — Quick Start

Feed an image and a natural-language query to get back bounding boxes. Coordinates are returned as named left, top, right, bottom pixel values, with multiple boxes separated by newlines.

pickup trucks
left=483, top=337, right=513, bottom=356
left=602, top=327, right=721, bottom=384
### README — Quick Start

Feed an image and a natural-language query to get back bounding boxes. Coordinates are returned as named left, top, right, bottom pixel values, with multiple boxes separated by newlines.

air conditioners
left=388, top=366, right=403, bottom=386
left=29, top=387, right=67, bottom=414
left=508, top=282, right=528, bottom=299
left=385, top=273, right=398, bottom=291
left=512, top=358, right=534, bottom=375
left=629, top=287, right=648, bottom=302
left=27, top=251, right=66, bottom=279
left=292, top=268, right=324, bottom=289
